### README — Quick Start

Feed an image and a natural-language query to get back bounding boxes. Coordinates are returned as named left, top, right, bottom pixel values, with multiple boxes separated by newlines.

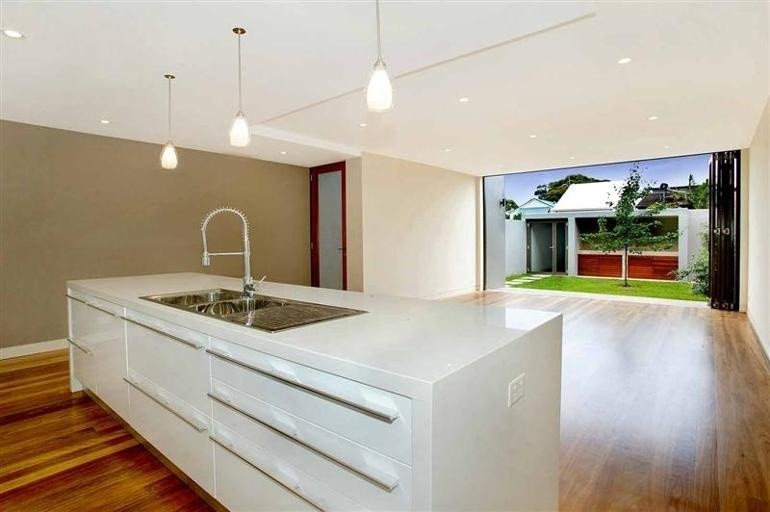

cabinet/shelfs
left=125, top=307, right=216, bottom=503
left=210, top=337, right=430, bottom=512
left=67, top=287, right=128, bottom=423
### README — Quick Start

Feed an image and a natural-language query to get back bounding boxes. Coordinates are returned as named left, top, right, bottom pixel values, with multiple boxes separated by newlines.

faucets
left=200, top=206, right=251, bottom=285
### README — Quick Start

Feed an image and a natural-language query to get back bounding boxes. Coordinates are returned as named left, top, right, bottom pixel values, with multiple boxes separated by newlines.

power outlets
left=506, top=373, right=527, bottom=408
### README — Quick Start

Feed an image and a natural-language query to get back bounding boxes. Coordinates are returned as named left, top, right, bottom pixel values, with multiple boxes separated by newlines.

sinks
left=138, top=288, right=246, bottom=305
left=187, top=298, right=290, bottom=316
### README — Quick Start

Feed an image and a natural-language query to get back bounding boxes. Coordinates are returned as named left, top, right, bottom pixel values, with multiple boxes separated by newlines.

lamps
left=160, top=74, right=178, bottom=169
left=366, top=0, right=392, bottom=111
left=229, top=25, right=248, bottom=147
